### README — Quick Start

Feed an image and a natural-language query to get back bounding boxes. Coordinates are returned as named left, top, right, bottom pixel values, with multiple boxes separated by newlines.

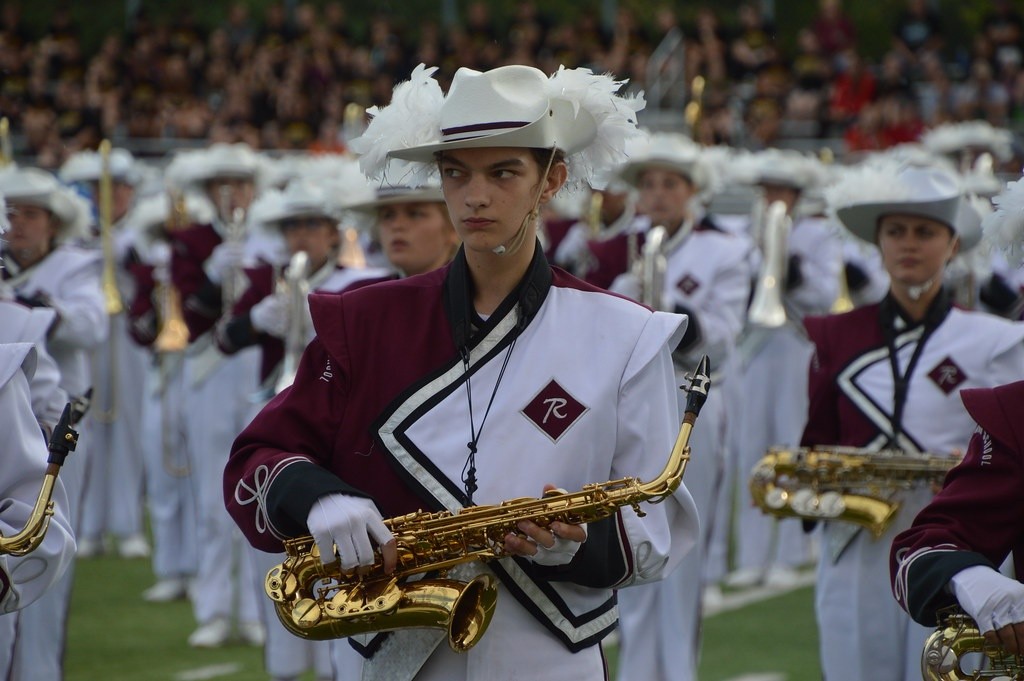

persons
left=794, top=153, right=1024, bottom=681
left=889, top=380, right=1024, bottom=655
left=0, top=169, right=112, bottom=681
left=0, top=0, right=1024, bottom=181
left=62, top=133, right=1024, bottom=681
left=352, top=63, right=460, bottom=278
left=222, top=65, right=703, bottom=681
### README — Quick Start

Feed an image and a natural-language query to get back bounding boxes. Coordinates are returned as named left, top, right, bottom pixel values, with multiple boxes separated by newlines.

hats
left=0, top=120, right=1024, bottom=287
left=826, top=145, right=984, bottom=257
left=348, top=60, right=647, bottom=191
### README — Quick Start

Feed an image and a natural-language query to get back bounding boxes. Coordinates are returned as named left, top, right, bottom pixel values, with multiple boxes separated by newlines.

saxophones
left=749, top=445, right=967, bottom=541
left=921, top=613, right=1024, bottom=681
left=263, top=352, right=713, bottom=656
left=1, top=400, right=79, bottom=560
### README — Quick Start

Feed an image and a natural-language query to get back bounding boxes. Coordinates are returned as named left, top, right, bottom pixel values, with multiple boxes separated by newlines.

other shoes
left=239, top=619, right=267, bottom=646
left=189, top=616, right=232, bottom=649
left=701, top=562, right=804, bottom=612
left=144, top=573, right=194, bottom=603
left=73, top=535, right=102, bottom=559
left=120, top=533, right=150, bottom=557
